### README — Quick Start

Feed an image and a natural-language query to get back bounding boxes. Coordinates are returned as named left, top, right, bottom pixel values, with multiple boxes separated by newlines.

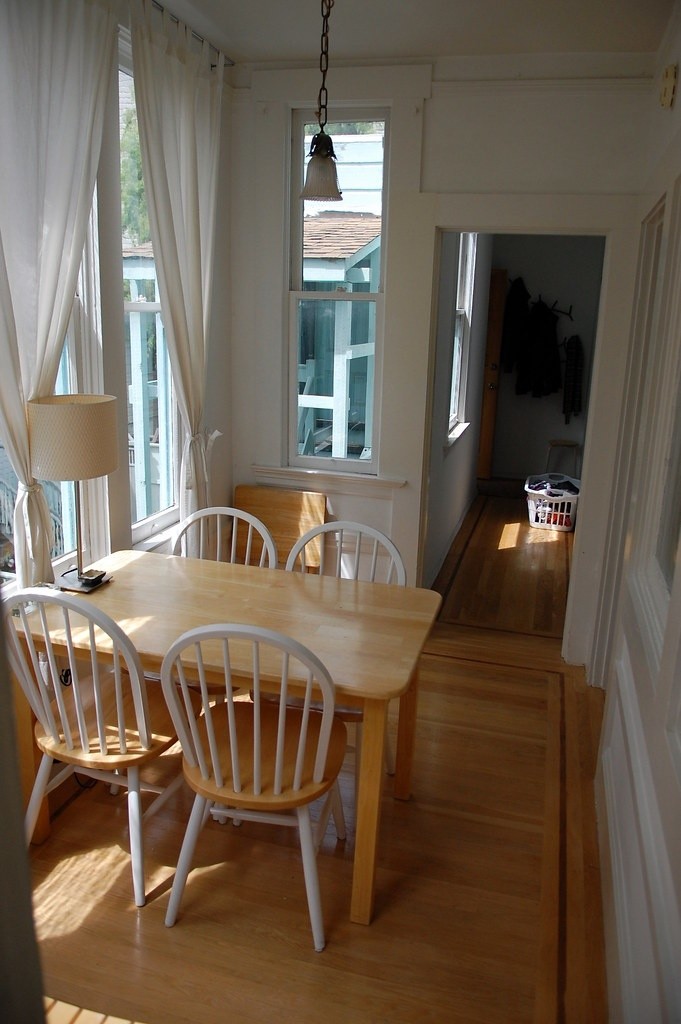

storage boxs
left=524, top=473, right=580, bottom=531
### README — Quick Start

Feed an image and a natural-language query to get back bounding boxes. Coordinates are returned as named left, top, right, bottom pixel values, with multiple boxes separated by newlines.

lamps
left=298, top=0, right=343, bottom=201
left=27, top=393, right=121, bottom=595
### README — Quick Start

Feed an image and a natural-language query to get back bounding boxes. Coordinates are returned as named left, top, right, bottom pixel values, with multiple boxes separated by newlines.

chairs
left=251, top=521, right=407, bottom=795
left=162, top=623, right=346, bottom=951
left=229, top=484, right=326, bottom=575
left=2, top=587, right=203, bottom=907
left=109, top=506, right=276, bottom=796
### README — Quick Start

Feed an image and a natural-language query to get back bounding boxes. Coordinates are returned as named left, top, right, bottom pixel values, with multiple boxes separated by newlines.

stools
left=545, top=440, right=580, bottom=477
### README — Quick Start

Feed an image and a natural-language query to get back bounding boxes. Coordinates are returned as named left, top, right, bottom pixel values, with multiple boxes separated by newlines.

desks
left=5, top=550, right=441, bottom=927
